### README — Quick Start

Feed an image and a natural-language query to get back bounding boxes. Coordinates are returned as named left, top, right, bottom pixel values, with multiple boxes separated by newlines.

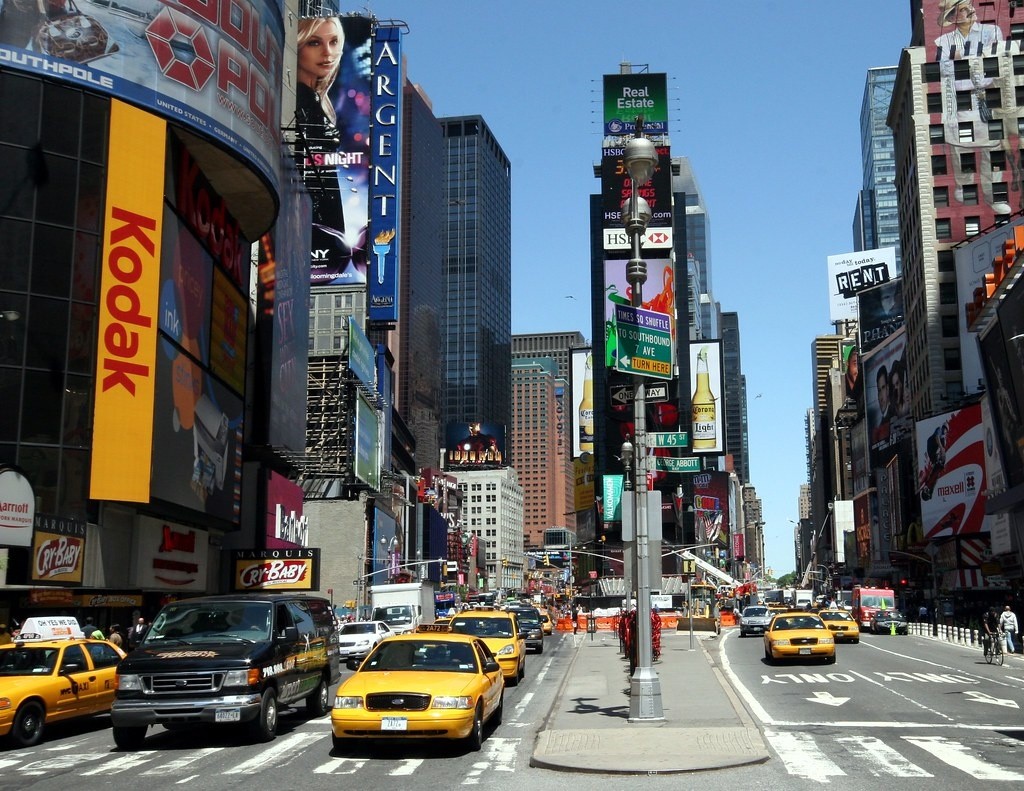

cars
left=871, top=610, right=908, bottom=635
left=330, top=593, right=553, bottom=752
left=0, top=617, right=128, bottom=746
left=737, top=600, right=859, bottom=665
left=339, top=621, right=396, bottom=658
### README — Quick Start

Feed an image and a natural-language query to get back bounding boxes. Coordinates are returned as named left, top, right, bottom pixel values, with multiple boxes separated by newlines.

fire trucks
left=850, top=584, right=896, bottom=629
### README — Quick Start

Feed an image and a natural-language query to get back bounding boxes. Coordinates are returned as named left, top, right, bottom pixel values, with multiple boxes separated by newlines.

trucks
left=371, top=582, right=435, bottom=636
left=758, top=582, right=853, bottom=607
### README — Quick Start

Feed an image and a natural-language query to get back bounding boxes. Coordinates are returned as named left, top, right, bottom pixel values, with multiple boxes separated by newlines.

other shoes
left=997, top=649, right=1000, bottom=654
left=984, top=651, right=987, bottom=655
left=1011, top=648, right=1015, bottom=653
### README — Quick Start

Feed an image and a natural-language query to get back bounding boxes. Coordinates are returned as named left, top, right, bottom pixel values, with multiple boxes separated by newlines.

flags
left=703, top=512, right=719, bottom=538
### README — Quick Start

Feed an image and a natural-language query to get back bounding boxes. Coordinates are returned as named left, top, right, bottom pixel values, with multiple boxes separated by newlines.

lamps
left=282, top=108, right=358, bottom=194
left=353, top=381, right=389, bottom=409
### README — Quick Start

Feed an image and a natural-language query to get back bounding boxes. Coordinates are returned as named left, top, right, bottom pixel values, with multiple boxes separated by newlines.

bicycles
left=984, top=632, right=1005, bottom=666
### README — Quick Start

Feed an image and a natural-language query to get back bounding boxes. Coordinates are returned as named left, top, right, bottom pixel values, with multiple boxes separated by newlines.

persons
left=295, top=17, right=350, bottom=287
left=576, top=604, right=582, bottom=613
left=845, top=346, right=858, bottom=396
left=130, top=617, right=148, bottom=648
left=934, top=0, right=1004, bottom=61
left=1000, top=606, right=1018, bottom=653
left=0, top=624, right=11, bottom=645
left=572, top=609, right=579, bottom=634
left=982, top=606, right=1002, bottom=655
left=80, top=617, right=98, bottom=639
left=919, top=604, right=927, bottom=623
left=105, top=623, right=122, bottom=648
left=877, top=359, right=904, bottom=426
left=654, top=604, right=659, bottom=613
left=91, top=622, right=107, bottom=640
left=340, top=614, right=354, bottom=623
left=924, top=421, right=949, bottom=494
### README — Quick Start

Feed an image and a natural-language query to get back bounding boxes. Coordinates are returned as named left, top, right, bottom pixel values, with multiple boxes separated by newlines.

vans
left=109, top=595, right=339, bottom=749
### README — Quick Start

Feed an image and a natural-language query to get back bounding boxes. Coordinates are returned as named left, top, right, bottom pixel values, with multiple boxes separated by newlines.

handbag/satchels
left=572, top=622, right=577, bottom=628
left=33, top=0, right=119, bottom=65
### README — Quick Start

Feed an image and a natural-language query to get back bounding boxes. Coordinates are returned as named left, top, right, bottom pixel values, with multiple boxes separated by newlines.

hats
left=1004, top=605, right=1011, bottom=610
left=938, top=0, right=970, bottom=27
left=91, top=630, right=105, bottom=640
left=0, top=624, right=7, bottom=628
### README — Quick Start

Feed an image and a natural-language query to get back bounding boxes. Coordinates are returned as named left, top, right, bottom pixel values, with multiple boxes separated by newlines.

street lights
left=622, top=137, right=668, bottom=726
left=387, top=535, right=398, bottom=584
left=623, top=434, right=634, bottom=613
left=731, top=521, right=766, bottom=583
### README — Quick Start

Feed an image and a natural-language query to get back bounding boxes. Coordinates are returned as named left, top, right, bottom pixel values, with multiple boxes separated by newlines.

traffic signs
left=604, top=384, right=668, bottom=407
left=614, top=303, right=673, bottom=382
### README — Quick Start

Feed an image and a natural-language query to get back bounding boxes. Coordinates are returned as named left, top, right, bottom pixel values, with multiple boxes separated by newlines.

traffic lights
left=556, top=594, right=561, bottom=598
left=565, top=589, right=570, bottom=596
left=543, top=554, right=549, bottom=566
left=899, top=572, right=908, bottom=590
left=856, top=567, right=865, bottom=578
left=502, top=557, right=508, bottom=566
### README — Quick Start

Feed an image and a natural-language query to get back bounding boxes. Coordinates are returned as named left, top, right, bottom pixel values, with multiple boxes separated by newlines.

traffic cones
left=881, top=597, right=887, bottom=610
left=890, top=621, right=896, bottom=636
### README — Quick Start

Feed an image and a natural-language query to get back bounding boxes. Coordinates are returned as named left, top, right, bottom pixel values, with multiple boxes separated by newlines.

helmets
left=942, top=422, right=949, bottom=431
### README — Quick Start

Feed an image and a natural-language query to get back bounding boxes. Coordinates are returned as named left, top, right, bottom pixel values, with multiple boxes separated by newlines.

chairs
left=778, top=621, right=788, bottom=630
